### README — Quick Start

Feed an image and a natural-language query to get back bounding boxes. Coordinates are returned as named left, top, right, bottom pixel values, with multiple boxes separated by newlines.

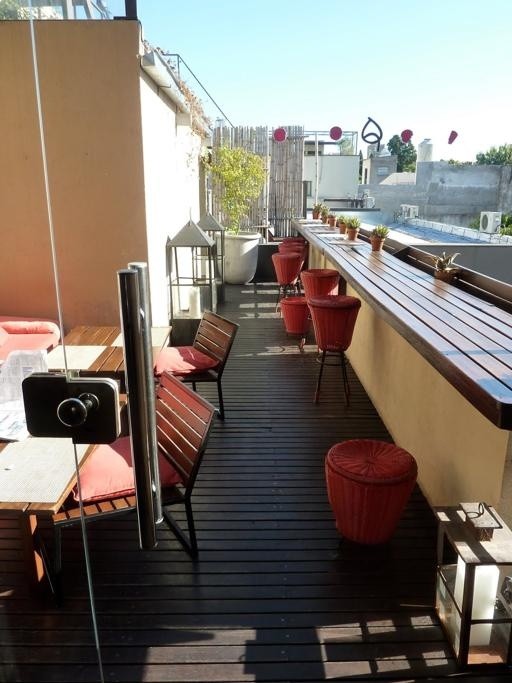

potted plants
left=370, top=223, right=389, bottom=251
left=345, top=216, right=360, bottom=240
left=434, top=251, right=462, bottom=283
left=200, top=143, right=266, bottom=285
left=328, top=211, right=337, bottom=227
left=312, top=202, right=322, bottom=219
left=321, top=205, right=330, bottom=223
left=338, top=216, right=348, bottom=233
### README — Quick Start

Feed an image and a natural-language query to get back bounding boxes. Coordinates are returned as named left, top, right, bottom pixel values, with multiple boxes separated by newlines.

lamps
left=431, top=502, right=511, bottom=673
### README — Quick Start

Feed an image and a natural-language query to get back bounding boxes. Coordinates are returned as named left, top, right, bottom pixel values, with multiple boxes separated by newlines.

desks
left=0, top=322, right=174, bottom=596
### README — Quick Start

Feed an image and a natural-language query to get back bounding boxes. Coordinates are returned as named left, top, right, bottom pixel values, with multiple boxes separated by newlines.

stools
left=280, top=297, right=309, bottom=336
left=307, top=295, right=361, bottom=407
left=272, top=252, right=306, bottom=312
left=324, top=440, right=417, bottom=545
left=0, top=316, right=60, bottom=362
left=278, top=236, right=308, bottom=297
left=299, top=268, right=340, bottom=321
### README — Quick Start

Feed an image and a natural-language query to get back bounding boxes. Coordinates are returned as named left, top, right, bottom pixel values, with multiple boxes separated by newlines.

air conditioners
left=363, top=196, right=375, bottom=208
left=407, top=205, right=420, bottom=218
left=399, top=203, right=409, bottom=217
left=479, top=211, right=502, bottom=233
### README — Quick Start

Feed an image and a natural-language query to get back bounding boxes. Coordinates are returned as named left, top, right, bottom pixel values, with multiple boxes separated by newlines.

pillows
left=155, top=345, right=221, bottom=376
left=70, top=436, right=185, bottom=507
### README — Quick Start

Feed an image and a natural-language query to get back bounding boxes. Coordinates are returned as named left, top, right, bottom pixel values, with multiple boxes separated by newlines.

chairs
left=151, top=308, right=240, bottom=421
left=45, top=371, right=218, bottom=599
